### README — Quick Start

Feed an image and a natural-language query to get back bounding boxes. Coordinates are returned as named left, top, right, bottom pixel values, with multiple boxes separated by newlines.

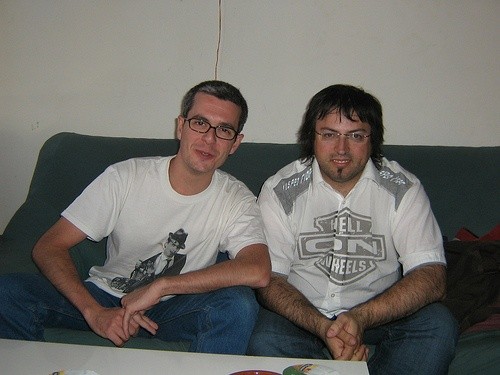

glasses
left=184, top=117, right=238, bottom=141
left=314, top=129, right=371, bottom=144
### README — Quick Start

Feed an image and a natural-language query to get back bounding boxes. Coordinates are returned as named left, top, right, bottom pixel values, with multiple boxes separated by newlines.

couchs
left=0, top=133, right=500, bottom=375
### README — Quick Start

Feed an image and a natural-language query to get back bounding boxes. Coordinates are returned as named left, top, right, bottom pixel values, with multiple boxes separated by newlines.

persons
left=247, top=84, right=458, bottom=375
left=0, top=80, right=272, bottom=356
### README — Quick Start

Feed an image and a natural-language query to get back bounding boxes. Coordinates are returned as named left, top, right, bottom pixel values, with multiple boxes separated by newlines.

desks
left=0, top=338, right=370, bottom=375
